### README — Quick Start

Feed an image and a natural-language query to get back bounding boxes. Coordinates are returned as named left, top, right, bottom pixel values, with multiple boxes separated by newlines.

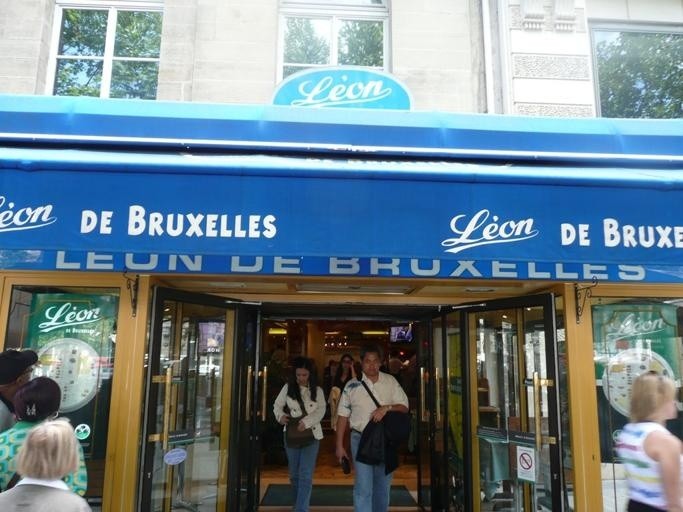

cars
left=199, top=356, right=220, bottom=377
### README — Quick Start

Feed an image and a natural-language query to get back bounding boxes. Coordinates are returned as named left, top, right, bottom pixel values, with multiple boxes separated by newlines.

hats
left=0, top=348, right=37, bottom=386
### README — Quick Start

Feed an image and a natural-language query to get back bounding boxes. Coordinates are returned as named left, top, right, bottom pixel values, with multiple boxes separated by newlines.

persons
left=333, top=346, right=412, bottom=512
left=270, top=357, right=328, bottom=512
left=0, top=346, right=42, bottom=431
left=321, top=352, right=420, bottom=456
left=617, top=370, right=683, bottom=512
left=0, top=415, right=101, bottom=511
left=0, top=377, right=91, bottom=497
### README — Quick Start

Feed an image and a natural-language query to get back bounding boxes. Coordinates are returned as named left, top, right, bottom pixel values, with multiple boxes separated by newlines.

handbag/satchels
left=356, top=410, right=411, bottom=477
left=283, top=416, right=316, bottom=450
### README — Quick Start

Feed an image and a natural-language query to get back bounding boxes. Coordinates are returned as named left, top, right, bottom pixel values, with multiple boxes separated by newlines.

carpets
left=259, top=484, right=418, bottom=506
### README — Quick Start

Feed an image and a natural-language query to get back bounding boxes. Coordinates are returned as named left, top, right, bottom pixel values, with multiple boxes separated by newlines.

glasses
left=342, top=359, right=351, bottom=364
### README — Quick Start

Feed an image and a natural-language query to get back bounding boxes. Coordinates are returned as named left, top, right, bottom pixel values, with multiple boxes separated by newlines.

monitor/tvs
left=388, top=324, right=413, bottom=342
left=198, top=320, right=224, bottom=356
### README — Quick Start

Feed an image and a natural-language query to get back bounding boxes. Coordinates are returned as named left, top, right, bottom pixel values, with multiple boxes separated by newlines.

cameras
left=342, top=456, right=351, bottom=475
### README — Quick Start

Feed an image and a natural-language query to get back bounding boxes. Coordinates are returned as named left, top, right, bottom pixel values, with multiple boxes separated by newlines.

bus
left=33, top=353, right=113, bottom=507
